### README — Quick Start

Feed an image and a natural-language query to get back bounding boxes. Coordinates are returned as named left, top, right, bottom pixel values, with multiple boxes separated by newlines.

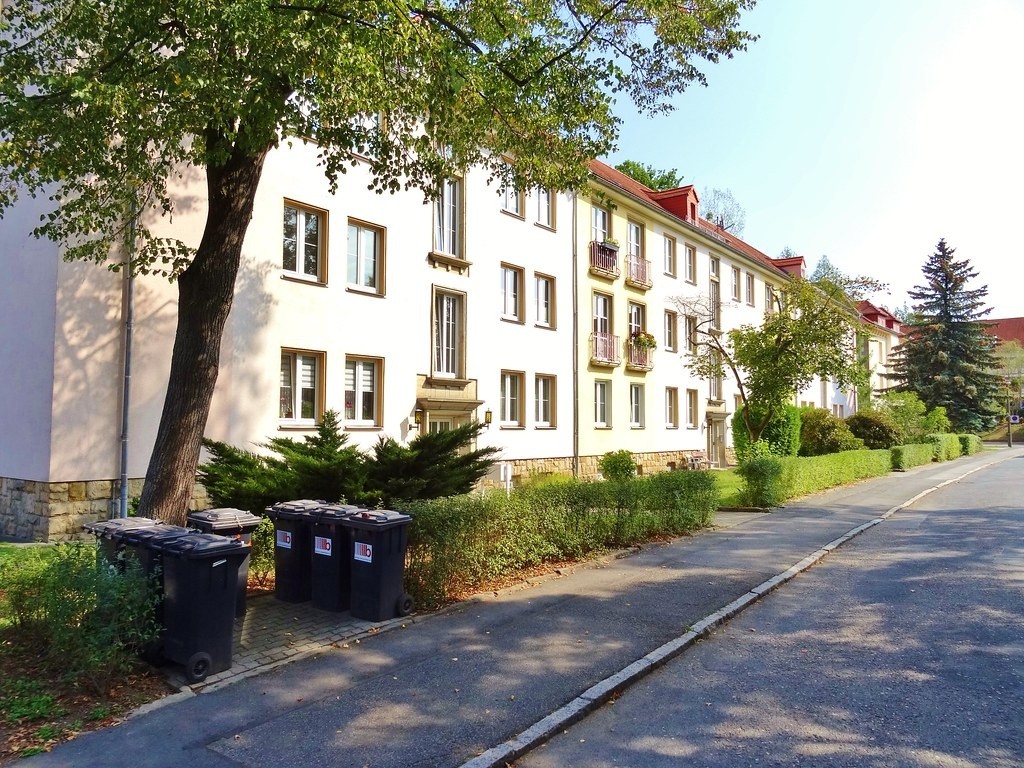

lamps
left=409, top=407, right=424, bottom=431
left=482, top=407, right=492, bottom=430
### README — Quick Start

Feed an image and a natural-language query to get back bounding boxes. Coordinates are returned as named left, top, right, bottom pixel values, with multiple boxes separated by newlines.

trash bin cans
left=83, top=517, right=165, bottom=618
left=151, top=533, right=252, bottom=684
left=110, top=524, right=199, bottom=658
left=340, top=509, right=414, bottom=623
left=186, top=507, right=260, bottom=619
left=264, top=499, right=329, bottom=605
left=301, top=504, right=369, bottom=614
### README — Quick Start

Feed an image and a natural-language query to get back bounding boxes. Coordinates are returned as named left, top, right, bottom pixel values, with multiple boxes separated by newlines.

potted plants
left=600, top=237, right=621, bottom=252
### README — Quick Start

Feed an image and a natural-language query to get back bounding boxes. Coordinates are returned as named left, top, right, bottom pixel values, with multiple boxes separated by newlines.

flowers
left=630, top=331, right=657, bottom=351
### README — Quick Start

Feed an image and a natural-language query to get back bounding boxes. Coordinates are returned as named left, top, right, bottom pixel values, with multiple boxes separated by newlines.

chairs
left=681, top=452, right=714, bottom=469
